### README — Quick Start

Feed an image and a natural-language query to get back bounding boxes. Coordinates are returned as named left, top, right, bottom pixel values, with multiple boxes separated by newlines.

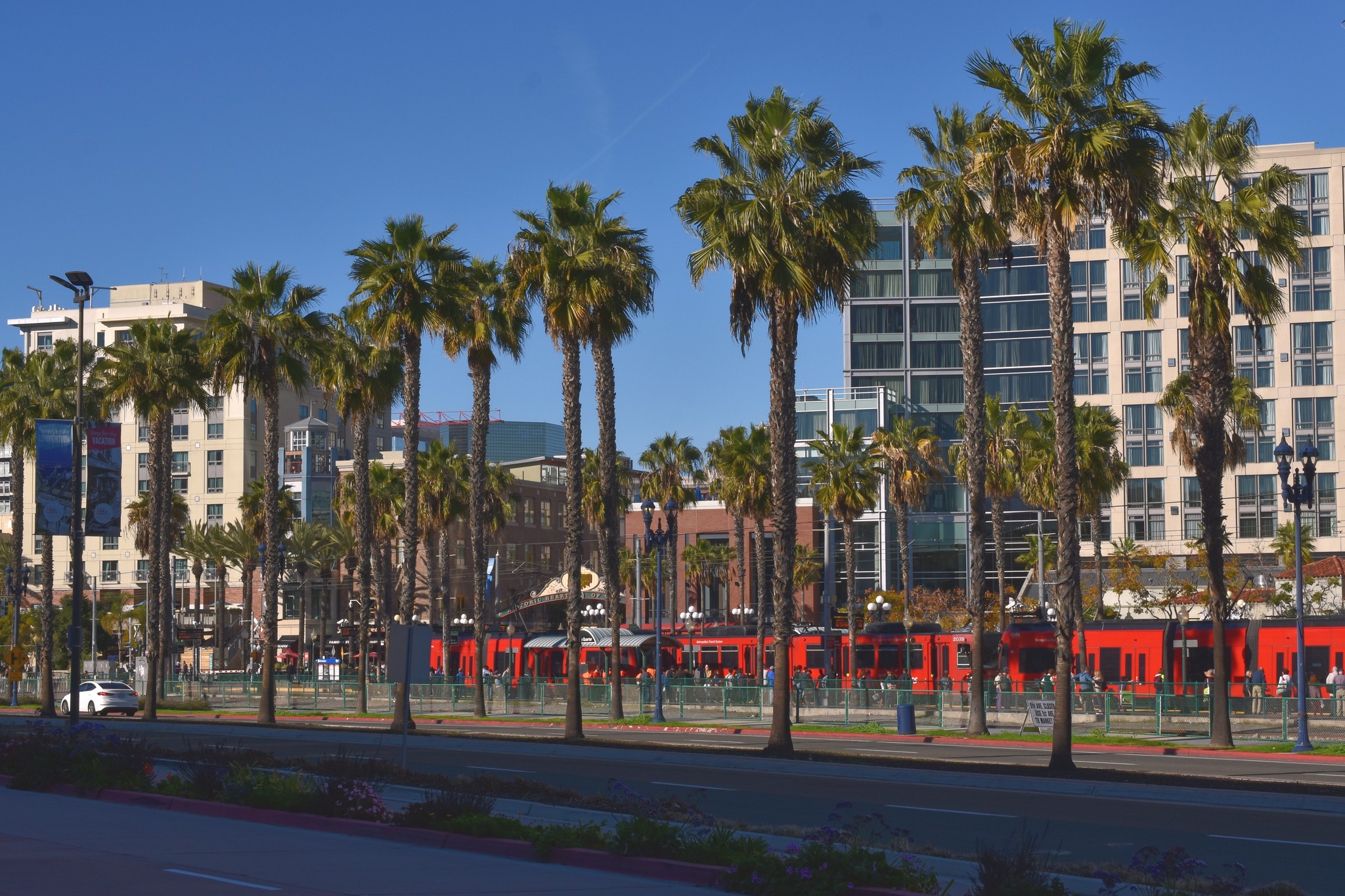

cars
left=59, top=681, right=142, bottom=716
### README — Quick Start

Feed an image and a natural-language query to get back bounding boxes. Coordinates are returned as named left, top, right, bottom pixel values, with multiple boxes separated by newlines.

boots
left=1313, top=706, right=1317, bottom=716
left=1319, top=706, right=1323, bottom=716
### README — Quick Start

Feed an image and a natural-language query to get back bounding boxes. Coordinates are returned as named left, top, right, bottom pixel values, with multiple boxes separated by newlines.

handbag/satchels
left=1203, top=678, right=1209, bottom=703
left=809, top=682, right=814, bottom=688
left=1276, top=675, right=1287, bottom=695
left=872, top=693, right=880, bottom=701
left=816, top=681, right=820, bottom=689
left=887, top=677, right=896, bottom=690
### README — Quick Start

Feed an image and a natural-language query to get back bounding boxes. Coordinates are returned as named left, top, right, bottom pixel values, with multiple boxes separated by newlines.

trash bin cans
left=897, top=704, right=917, bottom=735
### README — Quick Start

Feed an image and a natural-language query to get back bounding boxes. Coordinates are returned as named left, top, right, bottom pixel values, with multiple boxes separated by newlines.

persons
left=83, top=670, right=90, bottom=679
left=962, top=667, right=1134, bottom=712
left=636, top=663, right=913, bottom=708
left=1203, top=670, right=1214, bottom=694
left=1244, top=667, right=1345, bottom=717
left=580, top=665, right=612, bottom=700
left=175, top=661, right=193, bottom=681
left=4, top=668, right=8, bottom=678
left=26, top=664, right=33, bottom=679
left=938, top=670, right=954, bottom=710
left=1154, top=668, right=1165, bottom=713
left=523, top=667, right=555, bottom=698
left=457, top=668, right=467, bottom=698
left=482, top=665, right=510, bottom=700
left=283, top=661, right=294, bottom=682
left=370, top=661, right=385, bottom=683
left=427, top=665, right=445, bottom=699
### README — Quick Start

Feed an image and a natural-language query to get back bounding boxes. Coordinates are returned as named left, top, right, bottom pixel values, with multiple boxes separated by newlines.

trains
left=427, top=623, right=1345, bottom=712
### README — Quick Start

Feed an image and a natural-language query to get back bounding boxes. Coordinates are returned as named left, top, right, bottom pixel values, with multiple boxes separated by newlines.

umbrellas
left=207, top=600, right=233, bottom=606
left=237, top=602, right=252, bottom=607
left=274, top=650, right=298, bottom=665
left=353, top=652, right=378, bottom=657
left=131, top=600, right=181, bottom=608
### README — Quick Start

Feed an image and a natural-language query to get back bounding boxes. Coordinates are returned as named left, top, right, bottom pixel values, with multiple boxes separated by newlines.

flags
left=83, top=421, right=122, bottom=537
left=33, top=418, right=74, bottom=536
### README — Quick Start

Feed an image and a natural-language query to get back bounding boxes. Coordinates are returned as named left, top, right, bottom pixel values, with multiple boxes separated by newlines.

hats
left=177, top=662, right=181, bottom=664
left=1339, top=668, right=1344, bottom=671
left=1258, top=667, right=1265, bottom=673
left=494, top=670, right=499, bottom=674
left=1204, top=670, right=1214, bottom=678
left=770, top=666, right=775, bottom=670
left=1003, top=667, right=1011, bottom=670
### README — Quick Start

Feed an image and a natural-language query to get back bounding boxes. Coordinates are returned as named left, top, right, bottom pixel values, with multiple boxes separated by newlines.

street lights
left=393, top=615, right=419, bottom=730
left=1177, top=604, right=1190, bottom=694
left=582, top=604, right=604, bottom=628
left=904, top=609, right=912, bottom=685
left=53, top=268, right=118, bottom=716
left=453, top=614, right=476, bottom=690
left=4, top=562, right=33, bottom=707
left=505, top=616, right=514, bottom=697
left=1272, top=432, right=1318, bottom=754
left=688, top=620, right=694, bottom=680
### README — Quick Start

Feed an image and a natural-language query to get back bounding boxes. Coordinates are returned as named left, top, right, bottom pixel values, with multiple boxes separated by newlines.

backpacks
left=738, top=673, right=746, bottom=687
left=902, top=674, right=910, bottom=689
left=821, top=672, right=832, bottom=688
left=857, top=679, right=863, bottom=687
left=1102, top=680, right=1106, bottom=689
left=1000, top=673, right=1008, bottom=691
left=434, top=673, right=441, bottom=683
left=1153, top=674, right=1163, bottom=690
left=789, top=677, right=796, bottom=685
